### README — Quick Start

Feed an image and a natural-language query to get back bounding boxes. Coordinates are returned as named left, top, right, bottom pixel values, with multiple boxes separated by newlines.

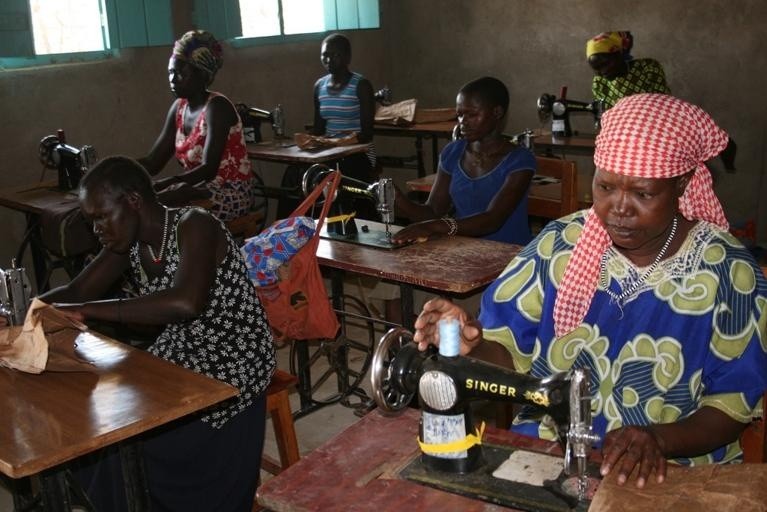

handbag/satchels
left=253, top=170, right=341, bottom=341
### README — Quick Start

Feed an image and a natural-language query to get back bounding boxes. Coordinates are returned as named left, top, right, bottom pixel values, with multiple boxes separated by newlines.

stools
left=249, top=369, right=300, bottom=511
left=226, top=212, right=265, bottom=246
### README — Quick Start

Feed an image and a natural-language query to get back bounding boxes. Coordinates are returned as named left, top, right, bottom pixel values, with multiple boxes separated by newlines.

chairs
left=739, top=265, right=767, bottom=464
left=525, top=157, right=578, bottom=219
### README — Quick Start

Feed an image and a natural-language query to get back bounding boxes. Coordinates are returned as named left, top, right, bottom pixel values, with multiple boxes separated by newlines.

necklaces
left=147, top=205, right=168, bottom=264
left=468, top=137, right=507, bottom=167
left=601, top=217, right=677, bottom=299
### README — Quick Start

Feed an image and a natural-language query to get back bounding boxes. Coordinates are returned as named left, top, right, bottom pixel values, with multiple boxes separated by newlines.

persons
left=587, top=28, right=671, bottom=132
left=413, top=93, right=767, bottom=490
left=389, top=77, right=538, bottom=246
left=0, top=156, right=277, bottom=512
left=277, top=33, right=378, bottom=219
left=133, top=29, right=256, bottom=222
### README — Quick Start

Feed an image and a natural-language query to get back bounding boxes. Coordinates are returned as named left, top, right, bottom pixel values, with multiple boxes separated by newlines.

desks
left=405, top=172, right=594, bottom=208
left=0, top=311, right=238, bottom=479
left=0, top=176, right=81, bottom=213
left=257, top=405, right=767, bottom=512
left=244, top=216, right=527, bottom=293
left=246, top=132, right=370, bottom=163
left=372, top=104, right=459, bottom=135
left=531, top=126, right=596, bottom=146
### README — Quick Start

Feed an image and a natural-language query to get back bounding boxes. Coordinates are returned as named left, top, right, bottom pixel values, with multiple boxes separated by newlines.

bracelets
left=118, top=298, right=123, bottom=325
left=440, top=217, right=458, bottom=237
left=173, top=175, right=181, bottom=182
